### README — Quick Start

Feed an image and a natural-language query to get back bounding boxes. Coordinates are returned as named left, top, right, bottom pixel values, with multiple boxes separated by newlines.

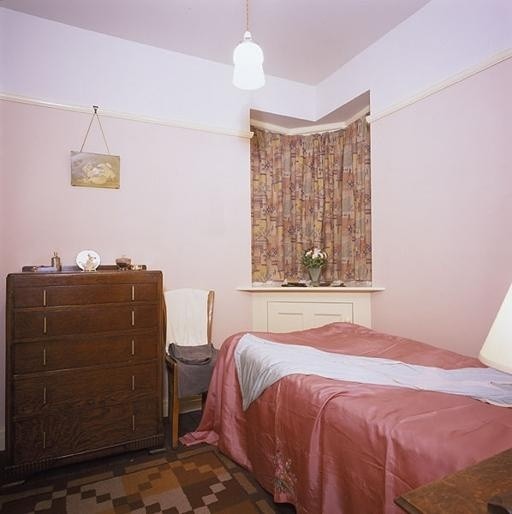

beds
left=180, top=323, right=512, bottom=514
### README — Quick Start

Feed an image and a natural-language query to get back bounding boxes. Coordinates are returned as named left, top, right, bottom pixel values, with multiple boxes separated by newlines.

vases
left=308, top=266, right=322, bottom=286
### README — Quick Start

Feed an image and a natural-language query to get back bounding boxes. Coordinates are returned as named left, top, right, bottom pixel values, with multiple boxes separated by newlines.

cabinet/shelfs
left=251, top=293, right=370, bottom=335
left=6, top=265, right=165, bottom=478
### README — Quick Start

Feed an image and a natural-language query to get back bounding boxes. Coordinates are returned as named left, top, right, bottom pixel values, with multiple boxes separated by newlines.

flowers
left=300, top=246, right=329, bottom=270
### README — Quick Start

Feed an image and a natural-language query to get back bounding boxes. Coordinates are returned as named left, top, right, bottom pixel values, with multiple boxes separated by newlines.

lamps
left=477, top=280, right=512, bottom=512
left=232, top=0, right=264, bottom=89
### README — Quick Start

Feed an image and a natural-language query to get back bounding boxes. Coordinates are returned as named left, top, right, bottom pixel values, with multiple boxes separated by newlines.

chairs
left=163, top=289, right=220, bottom=449
